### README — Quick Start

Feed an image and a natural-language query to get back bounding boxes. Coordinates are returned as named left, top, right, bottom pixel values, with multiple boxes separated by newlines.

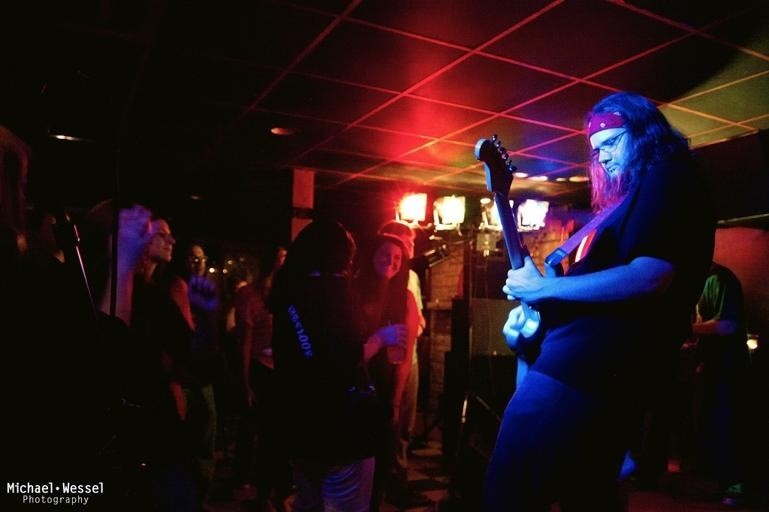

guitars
left=473, top=134, right=547, bottom=389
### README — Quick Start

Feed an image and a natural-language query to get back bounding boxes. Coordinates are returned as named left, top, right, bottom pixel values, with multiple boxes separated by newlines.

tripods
left=406, top=233, right=503, bottom=459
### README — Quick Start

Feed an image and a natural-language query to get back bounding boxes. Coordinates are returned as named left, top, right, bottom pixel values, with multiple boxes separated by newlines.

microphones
left=414, top=244, right=447, bottom=264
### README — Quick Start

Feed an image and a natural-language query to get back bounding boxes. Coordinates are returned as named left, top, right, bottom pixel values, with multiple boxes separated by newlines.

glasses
left=590, top=130, right=626, bottom=162
